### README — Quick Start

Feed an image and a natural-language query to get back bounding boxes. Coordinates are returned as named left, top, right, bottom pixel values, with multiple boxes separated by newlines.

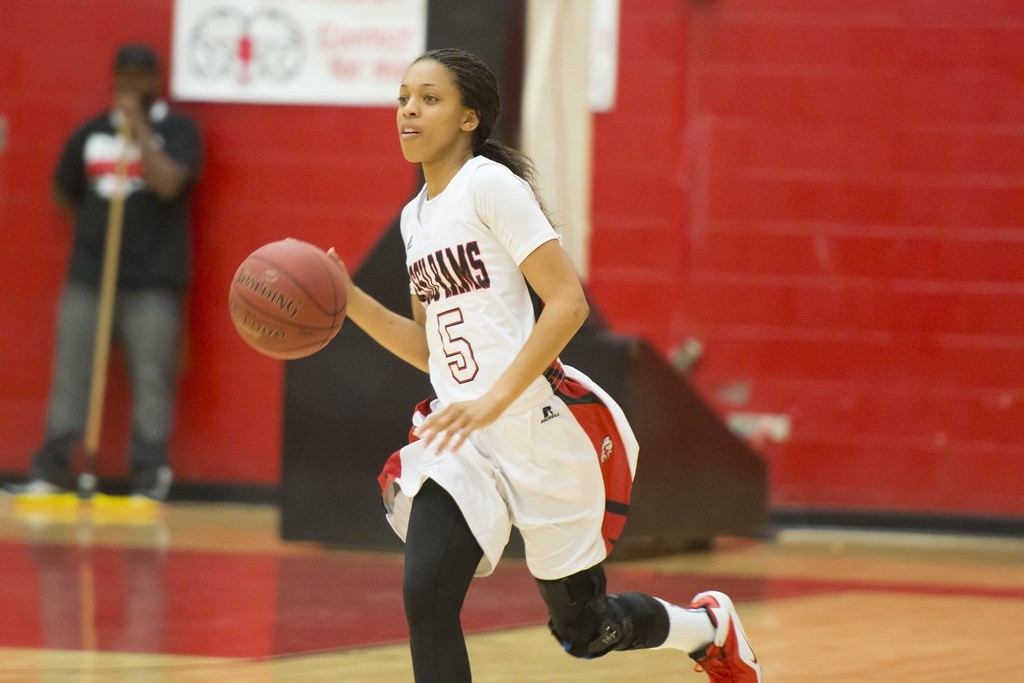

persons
left=4, top=44, right=203, bottom=502
left=283, top=49, right=762, bottom=683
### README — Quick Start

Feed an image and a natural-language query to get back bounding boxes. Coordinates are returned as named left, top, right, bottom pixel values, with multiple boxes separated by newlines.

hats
left=116, top=47, right=156, bottom=75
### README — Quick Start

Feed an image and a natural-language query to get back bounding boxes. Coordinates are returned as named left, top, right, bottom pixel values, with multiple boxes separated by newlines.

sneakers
left=689, top=591, right=761, bottom=683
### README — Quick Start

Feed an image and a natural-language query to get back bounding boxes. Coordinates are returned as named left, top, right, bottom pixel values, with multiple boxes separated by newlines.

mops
left=1, top=92, right=170, bottom=531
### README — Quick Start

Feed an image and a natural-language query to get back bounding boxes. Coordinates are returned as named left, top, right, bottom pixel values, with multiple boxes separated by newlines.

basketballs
left=227, top=239, right=349, bottom=362
